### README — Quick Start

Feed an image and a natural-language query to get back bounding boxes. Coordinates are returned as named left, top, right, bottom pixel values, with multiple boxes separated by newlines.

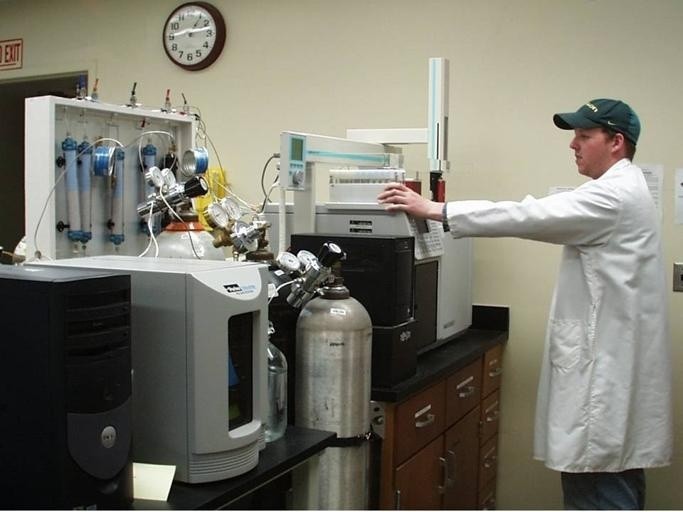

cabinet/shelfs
left=370, top=345, right=501, bottom=511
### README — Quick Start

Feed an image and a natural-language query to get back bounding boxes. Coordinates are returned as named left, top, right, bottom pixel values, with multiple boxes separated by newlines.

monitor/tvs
left=411, top=256, right=439, bottom=354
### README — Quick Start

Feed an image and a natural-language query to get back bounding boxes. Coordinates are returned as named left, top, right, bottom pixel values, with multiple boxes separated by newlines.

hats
left=551, top=97, right=642, bottom=147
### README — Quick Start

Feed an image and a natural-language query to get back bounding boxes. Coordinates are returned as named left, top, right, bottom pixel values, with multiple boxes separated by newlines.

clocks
left=160, top=1, right=225, bottom=71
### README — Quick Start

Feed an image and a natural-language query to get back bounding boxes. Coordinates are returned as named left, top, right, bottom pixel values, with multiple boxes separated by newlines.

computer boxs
left=290, top=233, right=419, bottom=387
left=0, top=263, right=132, bottom=510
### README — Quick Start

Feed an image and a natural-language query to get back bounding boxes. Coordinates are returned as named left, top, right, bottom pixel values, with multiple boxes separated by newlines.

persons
left=374, top=98, right=678, bottom=509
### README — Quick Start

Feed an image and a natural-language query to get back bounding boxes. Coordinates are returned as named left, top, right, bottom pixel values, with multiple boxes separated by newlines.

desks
left=117, top=422, right=337, bottom=509
